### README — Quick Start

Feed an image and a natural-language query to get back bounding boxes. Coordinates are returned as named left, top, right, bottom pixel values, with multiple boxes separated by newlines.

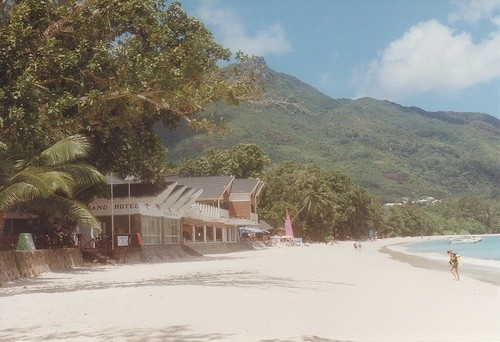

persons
left=287, top=234, right=376, bottom=252
left=240, top=234, right=273, bottom=247
left=449, top=253, right=460, bottom=280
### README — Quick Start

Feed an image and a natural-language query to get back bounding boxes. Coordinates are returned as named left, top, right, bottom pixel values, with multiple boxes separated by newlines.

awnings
left=238, top=226, right=264, bottom=233
